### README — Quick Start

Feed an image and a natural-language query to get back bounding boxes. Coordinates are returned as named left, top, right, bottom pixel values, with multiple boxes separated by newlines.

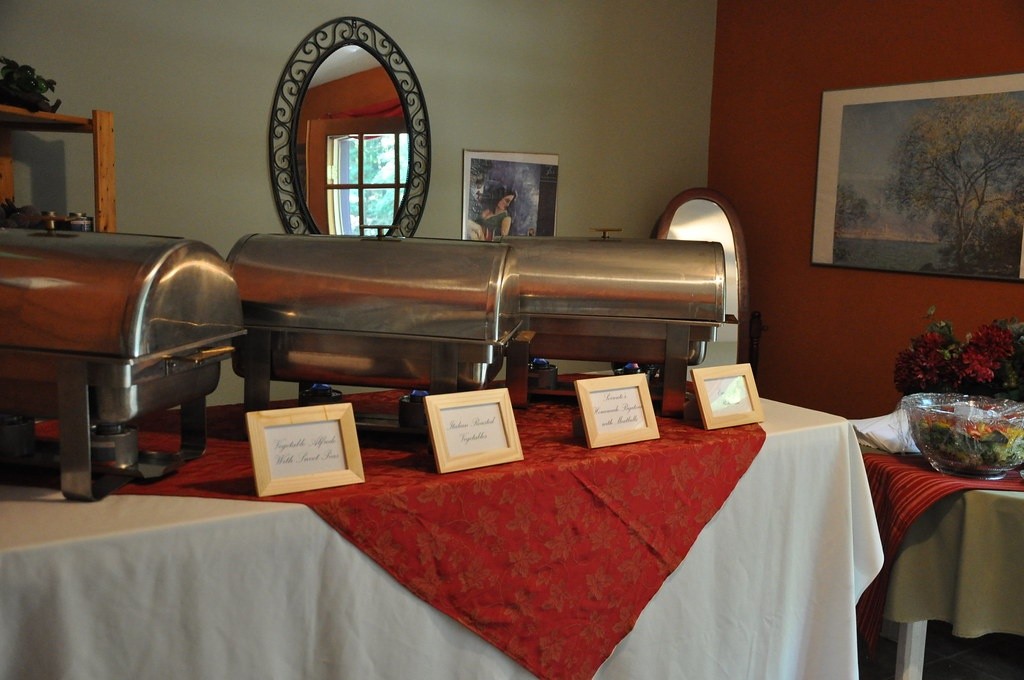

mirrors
left=263, top=14, right=436, bottom=234
left=653, top=188, right=753, bottom=381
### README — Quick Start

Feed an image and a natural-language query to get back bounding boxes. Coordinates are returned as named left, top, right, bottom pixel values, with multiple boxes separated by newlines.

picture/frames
left=574, top=371, right=661, bottom=450
left=808, top=70, right=1024, bottom=286
left=245, top=401, right=366, bottom=496
left=421, top=385, right=524, bottom=474
left=690, top=361, right=766, bottom=430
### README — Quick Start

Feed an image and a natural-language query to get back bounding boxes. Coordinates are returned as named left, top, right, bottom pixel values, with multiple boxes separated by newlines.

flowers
left=893, top=317, right=1013, bottom=394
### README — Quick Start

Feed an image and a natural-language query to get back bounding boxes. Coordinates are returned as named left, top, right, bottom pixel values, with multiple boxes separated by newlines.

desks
left=864, top=446, right=1024, bottom=680
left=0, top=397, right=890, bottom=680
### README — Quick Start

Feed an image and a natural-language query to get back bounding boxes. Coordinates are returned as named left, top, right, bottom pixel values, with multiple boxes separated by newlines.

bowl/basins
left=901, top=392, right=1024, bottom=481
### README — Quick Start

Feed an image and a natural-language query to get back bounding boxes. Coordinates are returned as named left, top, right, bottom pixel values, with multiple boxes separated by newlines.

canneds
left=69, top=217, right=94, bottom=231
